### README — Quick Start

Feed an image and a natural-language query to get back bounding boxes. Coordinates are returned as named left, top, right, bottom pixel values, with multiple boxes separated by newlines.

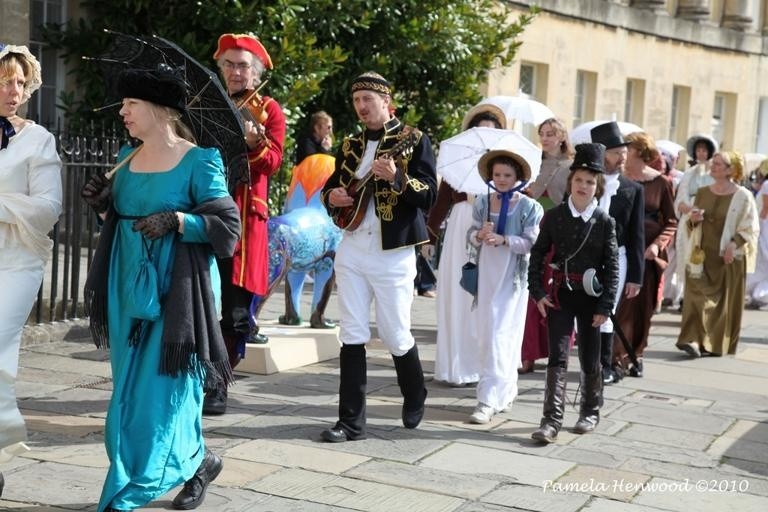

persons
left=302, top=113, right=767, bottom=375
left=462, top=163, right=543, bottom=422
left=0, top=54, right=63, bottom=445
left=82, top=100, right=243, bottom=511
left=204, top=35, right=286, bottom=413
left=321, top=92, right=437, bottom=443
left=529, top=171, right=622, bottom=445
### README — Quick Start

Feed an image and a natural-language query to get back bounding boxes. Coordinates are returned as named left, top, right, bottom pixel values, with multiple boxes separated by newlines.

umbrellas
left=476, top=92, right=553, bottom=130
left=82, top=28, right=253, bottom=192
left=441, top=127, right=543, bottom=237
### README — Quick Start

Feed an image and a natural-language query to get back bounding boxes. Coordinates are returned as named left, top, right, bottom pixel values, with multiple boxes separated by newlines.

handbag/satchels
left=689, top=245, right=705, bottom=265
left=459, top=262, right=478, bottom=294
left=123, top=232, right=162, bottom=322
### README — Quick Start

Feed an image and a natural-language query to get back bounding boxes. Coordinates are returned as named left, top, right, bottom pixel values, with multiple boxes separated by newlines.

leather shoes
left=203, top=387, right=227, bottom=414
left=321, top=426, right=348, bottom=442
left=743, top=298, right=765, bottom=310
left=173, top=443, right=223, bottom=510
left=602, top=365, right=642, bottom=385
left=402, top=402, right=425, bottom=428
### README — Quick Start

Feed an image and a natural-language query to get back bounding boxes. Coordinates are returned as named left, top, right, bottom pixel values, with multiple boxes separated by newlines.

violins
left=230, top=66, right=278, bottom=147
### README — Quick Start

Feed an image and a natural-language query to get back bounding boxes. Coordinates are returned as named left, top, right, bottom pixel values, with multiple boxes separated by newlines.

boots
left=531, top=366, right=567, bottom=444
left=575, top=363, right=604, bottom=432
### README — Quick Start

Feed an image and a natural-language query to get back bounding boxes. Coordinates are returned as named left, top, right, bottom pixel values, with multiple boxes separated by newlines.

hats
left=590, top=122, right=632, bottom=148
left=727, top=151, right=743, bottom=183
left=685, top=134, right=717, bottom=157
left=462, top=103, right=506, bottom=129
left=116, top=67, right=190, bottom=113
left=213, top=34, right=274, bottom=71
left=350, top=71, right=390, bottom=94
left=0, top=43, right=43, bottom=105
left=569, top=143, right=607, bottom=173
left=478, top=150, right=531, bottom=184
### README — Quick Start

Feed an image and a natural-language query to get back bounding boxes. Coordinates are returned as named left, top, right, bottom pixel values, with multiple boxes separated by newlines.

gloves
left=132, top=209, right=180, bottom=241
left=80, top=172, right=112, bottom=214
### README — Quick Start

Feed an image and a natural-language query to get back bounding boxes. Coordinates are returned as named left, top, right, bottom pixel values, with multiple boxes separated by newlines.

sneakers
left=676, top=341, right=701, bottom=356
left=469, top=401, right=497, bottom=423
